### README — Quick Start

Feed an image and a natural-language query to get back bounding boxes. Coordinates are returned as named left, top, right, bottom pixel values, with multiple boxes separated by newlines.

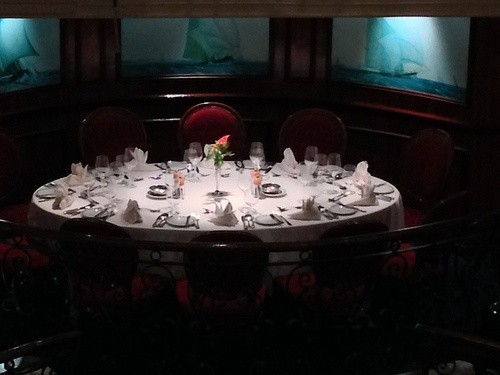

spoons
left=318, top=206, right=336, bottom=221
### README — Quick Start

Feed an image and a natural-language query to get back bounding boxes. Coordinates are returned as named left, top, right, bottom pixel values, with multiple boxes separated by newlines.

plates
left=369, top=181, right=395, bottom=193
left=326, top=204, right=357, bottom=215
left=36, top=187, right=66, bottom=199
left=168, top=216, right=197, bottom=226
left=255, top=214, right=284, bottom=226
left=81, top=208, right=112, bottom=218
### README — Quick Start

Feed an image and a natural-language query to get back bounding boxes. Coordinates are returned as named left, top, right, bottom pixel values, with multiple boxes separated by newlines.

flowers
left=204, top=134, right=235, bottom=165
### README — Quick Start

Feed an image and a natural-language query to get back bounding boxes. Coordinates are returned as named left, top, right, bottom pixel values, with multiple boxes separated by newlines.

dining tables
left=28, top=162, right=404, bottom=277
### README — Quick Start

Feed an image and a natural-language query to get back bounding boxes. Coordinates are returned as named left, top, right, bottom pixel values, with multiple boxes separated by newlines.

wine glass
left=185, top=143, right=203, bottom=181
left=327, top=153, right=342, bottom=194
left=95, top=155, right=109, bottom=197
left=168, top=185, right=184, bottom=216
left=304, top=146, right=320, bottom=187
left=249, top=141, right=263, bottom=175
left=236, top=175, right=257, bottom=214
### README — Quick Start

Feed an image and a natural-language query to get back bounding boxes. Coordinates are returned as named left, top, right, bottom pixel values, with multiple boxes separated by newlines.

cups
left=115, top=148, right=137, bottom=189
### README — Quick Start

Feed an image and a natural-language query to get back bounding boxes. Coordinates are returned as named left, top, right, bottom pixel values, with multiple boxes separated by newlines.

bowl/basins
left=261, top=183, right=282, bottom=194
left=146, top=184, right=169, bottom=199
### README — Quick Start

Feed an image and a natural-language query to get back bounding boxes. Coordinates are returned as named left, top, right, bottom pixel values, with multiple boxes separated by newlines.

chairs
left=0, top=102, right=456, bottom=331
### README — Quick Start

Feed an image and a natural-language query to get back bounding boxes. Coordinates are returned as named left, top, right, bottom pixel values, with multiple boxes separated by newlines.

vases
left=214, top=167, right=221, bottom=190
